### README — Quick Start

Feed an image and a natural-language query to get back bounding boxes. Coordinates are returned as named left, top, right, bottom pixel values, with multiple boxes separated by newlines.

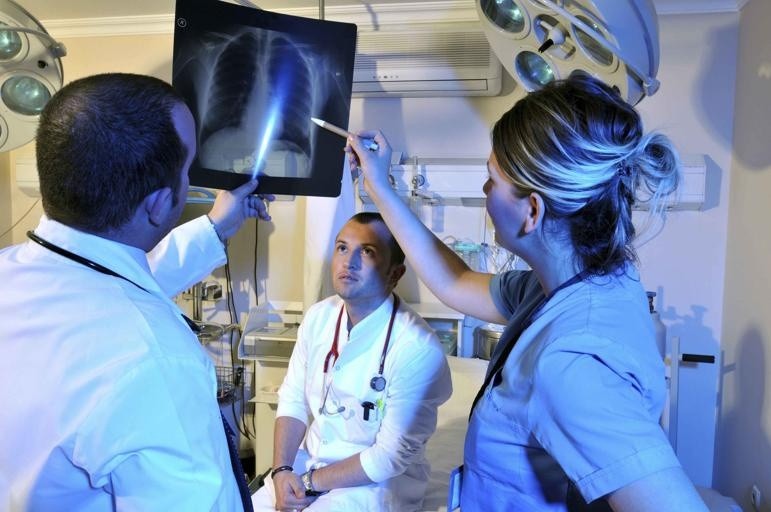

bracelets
left=207, top=217, right=225, bottom=246
left=299, top=468, right=330, bottom=496
left=270, top=465, right=293, bottom=479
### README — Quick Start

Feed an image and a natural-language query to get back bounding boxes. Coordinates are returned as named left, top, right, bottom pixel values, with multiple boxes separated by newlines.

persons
left=0, top=72, right=276, bottom=511
left=341, top=77, right=710, bottom=511
left=251, top=212, right=453, bottom=511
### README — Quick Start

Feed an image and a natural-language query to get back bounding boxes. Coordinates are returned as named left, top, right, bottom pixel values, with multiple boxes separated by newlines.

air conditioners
left=258, top=3, right=504, bottom=98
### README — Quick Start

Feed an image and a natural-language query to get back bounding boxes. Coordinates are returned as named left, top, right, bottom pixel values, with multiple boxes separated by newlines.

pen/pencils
left=361, top=400, right=383, bottom=421
left=310, top=117, right=380, bottom=151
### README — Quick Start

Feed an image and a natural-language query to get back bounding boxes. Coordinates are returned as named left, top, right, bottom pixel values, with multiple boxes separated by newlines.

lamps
left=472, top=0, right=661, bottom=110
left=1, top=0, right=67, bottom=153
